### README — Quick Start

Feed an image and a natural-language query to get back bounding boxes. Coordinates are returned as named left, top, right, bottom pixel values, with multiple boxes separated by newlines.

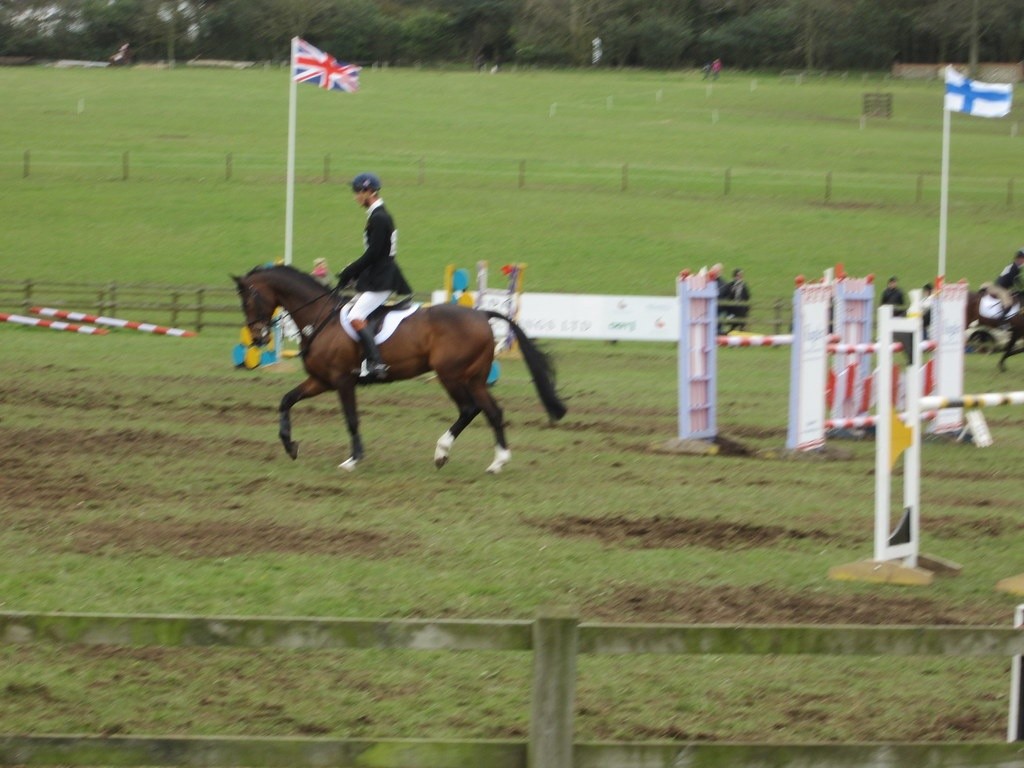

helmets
left=1015, top=251, right=1024, bottom=258
left=348, top=174, right=380, bottom=192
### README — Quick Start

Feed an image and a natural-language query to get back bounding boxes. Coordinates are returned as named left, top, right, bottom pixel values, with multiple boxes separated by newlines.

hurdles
left=655, top=260, right=969, bottom=466
left=827, top=304, right=1024, bottom=596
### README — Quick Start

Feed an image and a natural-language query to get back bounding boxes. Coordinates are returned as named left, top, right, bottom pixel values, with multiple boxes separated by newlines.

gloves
left=336, top=275, right=347, bottom=292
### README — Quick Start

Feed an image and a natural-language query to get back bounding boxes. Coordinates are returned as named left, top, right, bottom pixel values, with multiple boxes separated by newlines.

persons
left=715, top=269, right=750, bottom=334
left=990, top=250, right=1024, bottom=318
left=921, top=282, right=932, bottom=340
left=339, top=172, right=411, bottom=374
left=882, top=277, right=904, bottom=316
left=313, top=258, right=327, bottom=277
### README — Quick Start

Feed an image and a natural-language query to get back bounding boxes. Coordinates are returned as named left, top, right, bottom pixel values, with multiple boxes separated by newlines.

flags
left=944, top=69, right=1012, bottom=117
left=293, top=39, right=362, bottom=92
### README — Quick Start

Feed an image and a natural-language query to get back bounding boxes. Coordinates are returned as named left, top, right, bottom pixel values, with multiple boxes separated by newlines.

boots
left=998, top=308, right=1012, bottom=323
left=356, top=327, right=379, bottom=375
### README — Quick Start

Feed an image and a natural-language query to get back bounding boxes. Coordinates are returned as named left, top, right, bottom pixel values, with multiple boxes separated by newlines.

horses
left=229, top=263, right=568, bottom=476
left=965, top=289, right=1024, bottom=373
